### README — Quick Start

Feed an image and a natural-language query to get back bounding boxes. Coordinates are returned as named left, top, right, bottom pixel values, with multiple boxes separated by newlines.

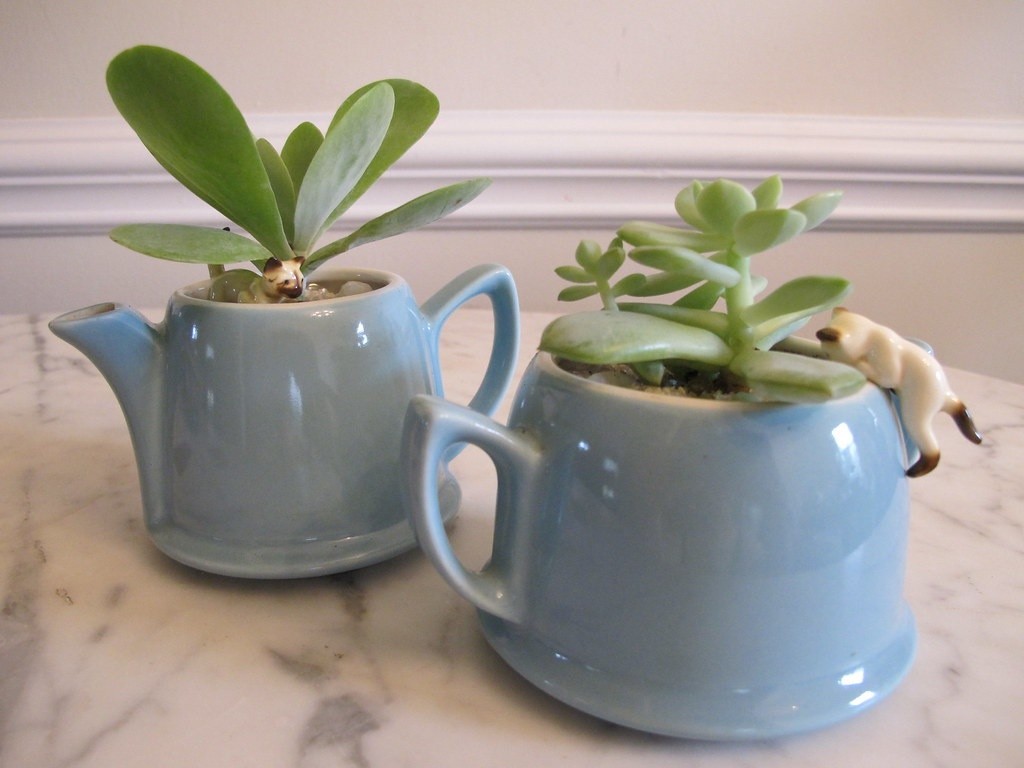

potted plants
left=399, top=175, right=984, bottom=746
left=42, top=43, right=527, bottom=579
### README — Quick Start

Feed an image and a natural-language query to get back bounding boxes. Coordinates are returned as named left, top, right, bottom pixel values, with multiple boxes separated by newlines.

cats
left=207, top=256, right=306, bottom=304
left=814, top=304, right=983, bottom=477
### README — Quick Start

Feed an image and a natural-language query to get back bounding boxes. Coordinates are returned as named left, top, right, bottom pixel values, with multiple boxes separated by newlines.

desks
left=0, top=307, right=1024, bottom=768
